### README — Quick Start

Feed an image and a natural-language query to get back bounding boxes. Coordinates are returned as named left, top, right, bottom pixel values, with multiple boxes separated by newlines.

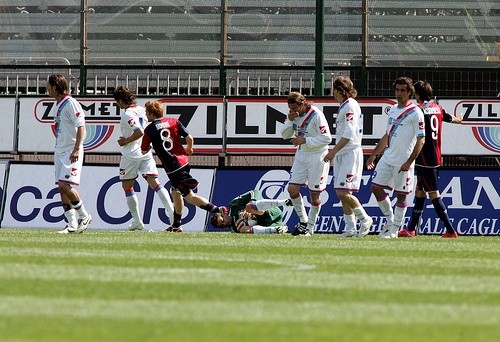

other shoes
left=129, top=221, right=144, bottom=230
left=359, top=216, right=373, bottom=237
left=276, top=226, right=288, bottom=234
left=346, top=224, right=355, bottom=236
left=285, top=198, right=293, bottom=207
left=163, top=226, right=182, bottom=232
left=219, top=206, right=227, bottom=214
left=378, top=225, right=398, bottom=238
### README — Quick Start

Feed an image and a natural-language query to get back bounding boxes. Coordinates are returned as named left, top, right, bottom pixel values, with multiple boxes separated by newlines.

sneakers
left=440, top=231, right=458, bottom=238
left=75, top=213, right=92, bottom=234
left=291, top=222, right=314, bottom=236
left=398, top=227, right=416, bottom=237
left=57, top=227, right=77, bottom=234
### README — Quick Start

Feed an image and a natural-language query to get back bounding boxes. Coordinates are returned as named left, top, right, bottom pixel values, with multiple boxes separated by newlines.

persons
left=366, top=78, right=425, bottom=238
left=211, top=190, right=293, bottom=233
left=141, top=100, right=227, bottom=232
left=113, top=86, right=174, bottom=231
left=281, top=92, right=332, bottom=236
left=47, top=75, right=92, bottom=234
left=324, top=76, right=373, bottom=237
left=398, top=80, right=463, bottom=238
